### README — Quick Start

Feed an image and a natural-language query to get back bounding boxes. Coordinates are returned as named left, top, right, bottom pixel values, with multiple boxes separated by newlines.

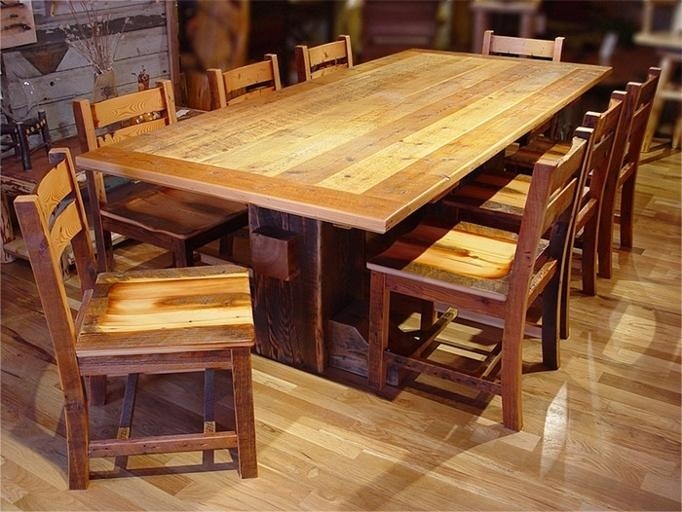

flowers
left=58, top=1, right=135, bottom=77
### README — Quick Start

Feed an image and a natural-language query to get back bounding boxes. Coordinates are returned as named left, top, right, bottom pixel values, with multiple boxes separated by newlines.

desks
left=634, top=31, right=682, bottom=153
left=0, top=105, right=209, bottom=280
left=76, top=48, right=614, bottom=384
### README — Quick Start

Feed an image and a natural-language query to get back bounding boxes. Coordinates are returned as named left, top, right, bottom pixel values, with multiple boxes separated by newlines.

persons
left=186, top=0, right=252, bottom=101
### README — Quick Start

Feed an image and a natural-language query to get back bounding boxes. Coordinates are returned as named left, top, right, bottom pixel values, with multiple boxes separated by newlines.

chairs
left=442, top=90, right=629, bottom=341
left=73, top=79, right=249, bottom=273
left=13, top=148, right=259, bottom=490
left=366, top=126, right=594, bottom=432
left=482, top=30, right=566, bottom=146
left=295, top=34, right=353, bottom=83
left=503, top=67, right=662, bottom=279
left=206, top=53, right=282, bottom=109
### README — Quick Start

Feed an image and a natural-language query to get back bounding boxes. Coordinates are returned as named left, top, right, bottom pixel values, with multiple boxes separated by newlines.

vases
left=92, top=69, right=127, bottom=134
